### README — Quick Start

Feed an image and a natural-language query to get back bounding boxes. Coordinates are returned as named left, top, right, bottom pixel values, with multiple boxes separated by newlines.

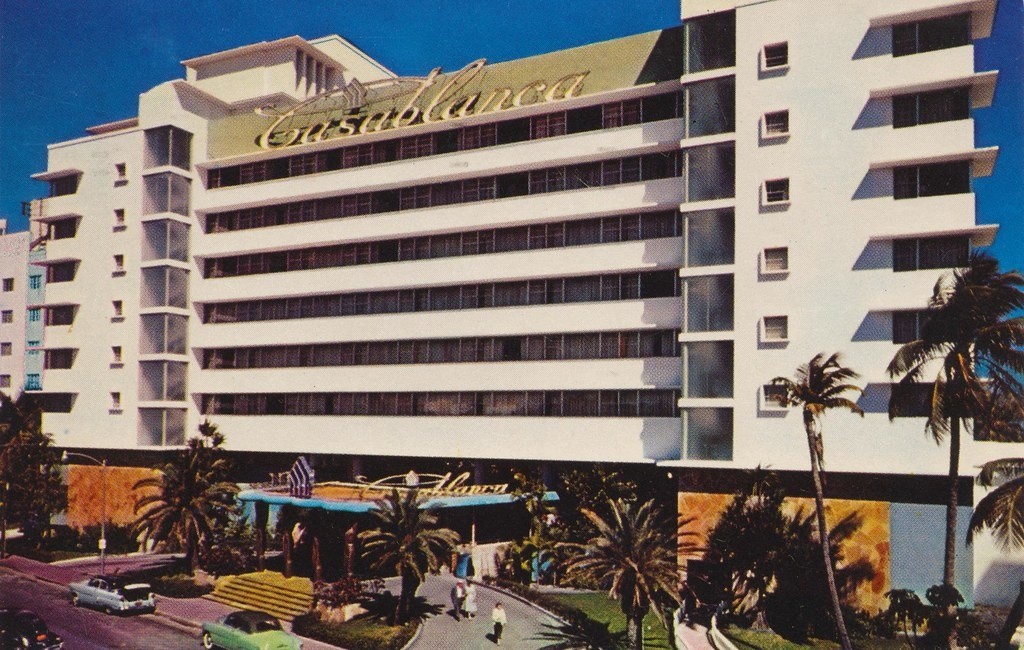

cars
left=0, top=608, right=65, bottom=648
left=202, top=608, right=304, bottom=649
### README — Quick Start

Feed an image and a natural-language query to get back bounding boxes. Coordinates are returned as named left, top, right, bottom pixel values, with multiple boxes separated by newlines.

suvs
left=69, top=574, right=156, bottom=615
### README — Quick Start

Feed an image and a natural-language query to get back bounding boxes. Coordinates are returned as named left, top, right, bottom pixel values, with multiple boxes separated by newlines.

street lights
left=61, top=451, right=106, bottom=575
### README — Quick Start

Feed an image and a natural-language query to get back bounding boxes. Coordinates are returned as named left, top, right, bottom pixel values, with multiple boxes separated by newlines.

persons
left=492, top=602, right=508, bottom=646
left=451, top=579, right=477, bottom=622
